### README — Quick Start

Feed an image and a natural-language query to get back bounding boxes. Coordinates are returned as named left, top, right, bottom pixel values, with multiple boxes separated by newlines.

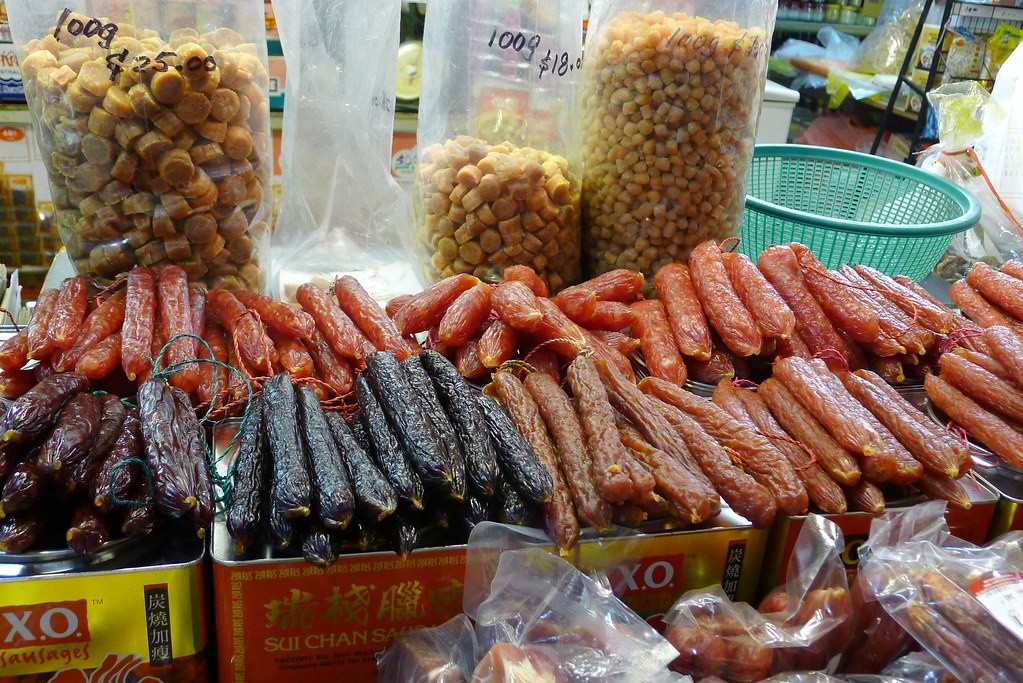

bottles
left=778, top=0, right=863, bottom=24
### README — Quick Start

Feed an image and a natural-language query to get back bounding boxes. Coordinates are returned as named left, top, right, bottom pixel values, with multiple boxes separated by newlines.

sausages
left=0, top=237, right=1023, bottom=683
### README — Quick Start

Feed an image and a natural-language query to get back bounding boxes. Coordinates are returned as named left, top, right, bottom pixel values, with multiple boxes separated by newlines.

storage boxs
left=0, top=0, right=1023, bottom=683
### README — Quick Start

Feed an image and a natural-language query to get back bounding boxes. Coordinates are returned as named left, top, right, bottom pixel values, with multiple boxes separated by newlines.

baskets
left=727, top=142, right=982, bottom=284
